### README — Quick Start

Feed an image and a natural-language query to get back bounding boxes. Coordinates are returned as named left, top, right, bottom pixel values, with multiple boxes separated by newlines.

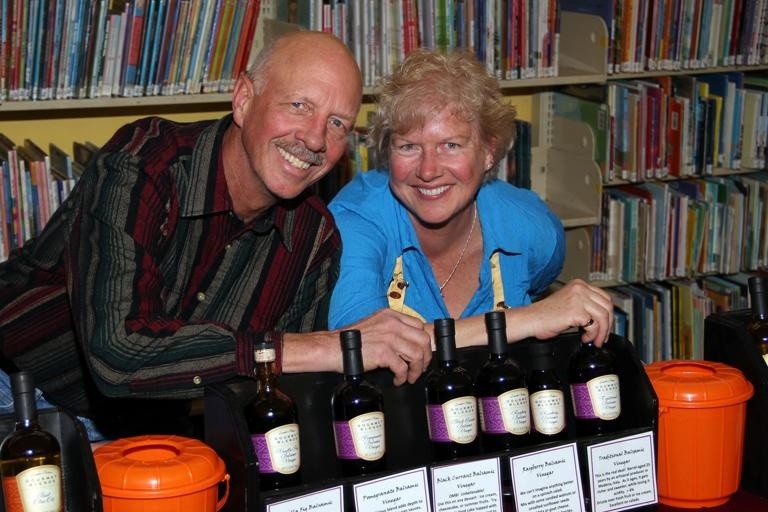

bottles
left=527, top=334, right=566, bottom=441
left=420, top=317, right=479, bottom=456
left=243, top=330, right=302, bottom=486
left=478, top=310, right=530, bottom=452
left=0, top=369, right=63, bottom=512
left=569, top=324, right=625, bottom=433
left=746, top=272, right=768, bottom=380
left=329, top=328, right=392, bottom=471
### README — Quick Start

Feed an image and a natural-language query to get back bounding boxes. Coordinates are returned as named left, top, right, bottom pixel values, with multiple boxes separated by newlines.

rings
left=580, top=318, right=595, bottom=332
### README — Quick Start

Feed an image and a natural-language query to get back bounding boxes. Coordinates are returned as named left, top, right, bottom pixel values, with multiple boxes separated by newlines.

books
left=1, top=133, right=104, bottom=259
left=556, top=1, right=768, bottom=74
left=0, top=0, right=562, bottom=101
left=551, top=271, right=768, bottom=365
left=551, top=72, right=768, bottom=183
left=586, top=170, right=768, bottom=284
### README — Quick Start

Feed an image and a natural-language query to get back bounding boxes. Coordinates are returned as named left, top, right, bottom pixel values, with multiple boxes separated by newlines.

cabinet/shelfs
left=0, top=328, right=661, bottom=511
left=1, top=0, right=606, bottom=305
left=700, top=304, right=767, bottom=495
left=545, top=1, right=768, bottom=355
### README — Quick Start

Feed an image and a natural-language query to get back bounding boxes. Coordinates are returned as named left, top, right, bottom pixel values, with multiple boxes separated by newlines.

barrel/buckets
left=92, top=435, right=231, bottom=512
left=644, top=357, right=756, bottom=510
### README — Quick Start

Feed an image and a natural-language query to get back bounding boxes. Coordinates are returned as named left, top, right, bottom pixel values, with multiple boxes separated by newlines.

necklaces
left=437, top=202, right=479, bottom=293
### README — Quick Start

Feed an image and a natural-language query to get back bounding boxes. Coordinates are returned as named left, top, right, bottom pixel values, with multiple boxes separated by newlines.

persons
left=1, top=27, right=434, bottom=445
left=319, top=45, right=618, bottom=350
